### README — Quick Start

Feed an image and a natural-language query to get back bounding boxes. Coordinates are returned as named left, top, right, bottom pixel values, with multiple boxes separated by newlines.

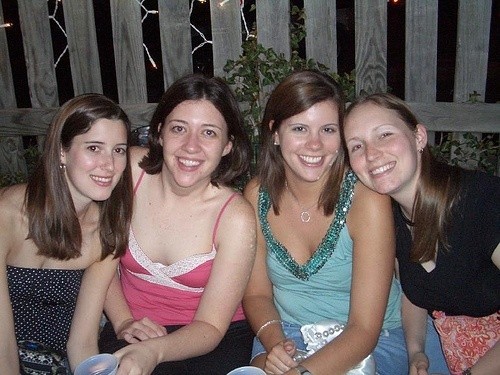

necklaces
left=396, top=205, right=417, bottom=227
left=283, top=179, right=321, bottom=223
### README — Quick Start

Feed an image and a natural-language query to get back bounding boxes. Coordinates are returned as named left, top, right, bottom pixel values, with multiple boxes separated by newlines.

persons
left=1, top=93, right=134, bottom=375
left=341, top=93, right=500, bottom=375
left=243, top=69, right=450, bottom=375
left=98, top=73, right=257, bottom=375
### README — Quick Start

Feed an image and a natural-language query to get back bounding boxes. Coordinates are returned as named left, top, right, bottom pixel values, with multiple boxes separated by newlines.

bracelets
left=256, top=319, right=285, bottom=341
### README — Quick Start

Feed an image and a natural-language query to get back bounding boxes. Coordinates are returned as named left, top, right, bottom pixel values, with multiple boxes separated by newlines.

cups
left=73, top=353, right=119, bottom=375
left=226, top=366, right=267, bottom=375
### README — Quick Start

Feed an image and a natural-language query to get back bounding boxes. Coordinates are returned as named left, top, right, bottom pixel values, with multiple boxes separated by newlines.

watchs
left=295, top=365, right=313, bottom=375
